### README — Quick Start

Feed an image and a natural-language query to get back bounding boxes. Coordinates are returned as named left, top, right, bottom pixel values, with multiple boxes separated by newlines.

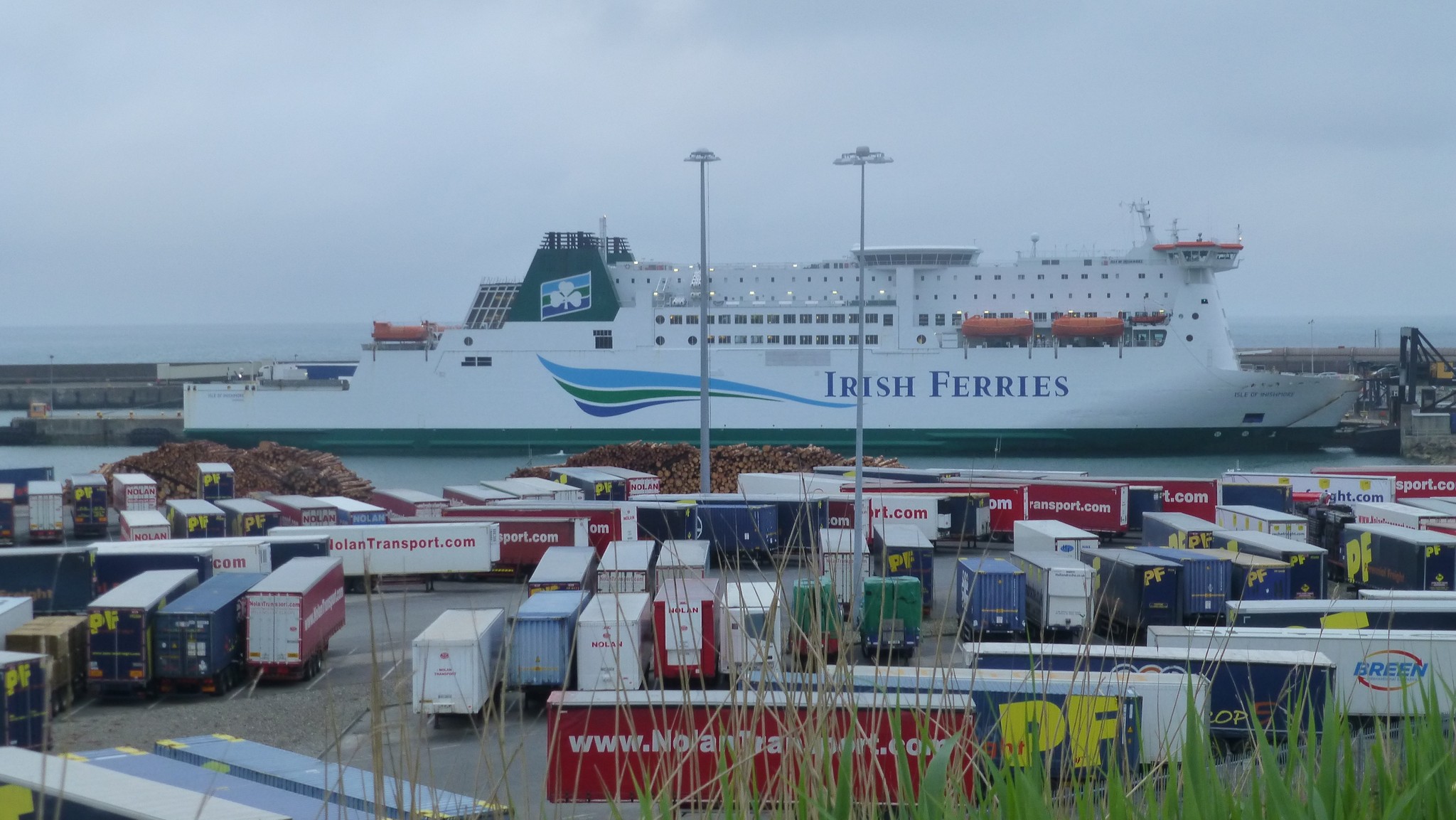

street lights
left=1307, top=319, right=1315, bottom=375
left=682, top=143, right=723, bottom=494
left=835, top=144, right=892, bottom=634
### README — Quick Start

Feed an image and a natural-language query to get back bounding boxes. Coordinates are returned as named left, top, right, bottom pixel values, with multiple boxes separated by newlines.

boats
left=1128, top=313, right=1166, bottom=323
left=1051, top=313, right=1125, bottom=338
left=370, top=319, right=445, bottom=343
left=961, top=314, right=1035, bottom=338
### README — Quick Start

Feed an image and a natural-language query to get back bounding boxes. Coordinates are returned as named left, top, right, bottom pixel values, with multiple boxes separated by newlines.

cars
left=1312, top=371, right=1340, bottom=379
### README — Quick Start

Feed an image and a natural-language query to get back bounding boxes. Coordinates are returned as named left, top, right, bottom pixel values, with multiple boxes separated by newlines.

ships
left=180, top=192, right=1407, bottom=462
